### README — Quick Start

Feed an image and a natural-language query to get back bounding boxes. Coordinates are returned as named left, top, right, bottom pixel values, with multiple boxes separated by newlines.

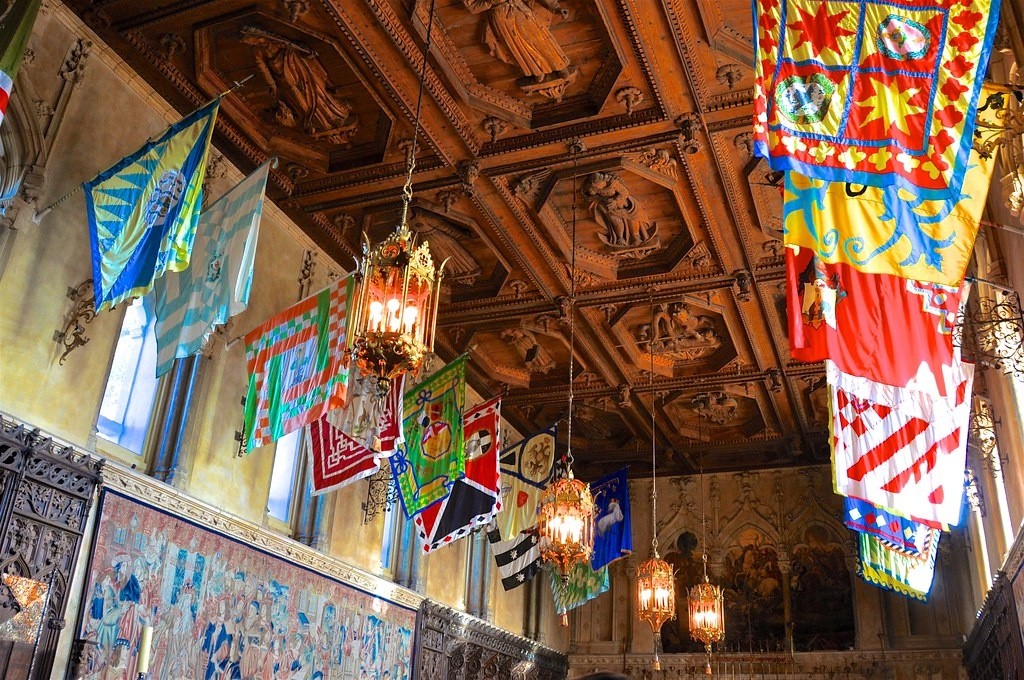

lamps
left=343, top=2, right=453, bottom=454
left=536, top=139, right=602, bottom=626
left=689, top=376, right=725, bottom=676
left=636, top=305, right=680, bottom=672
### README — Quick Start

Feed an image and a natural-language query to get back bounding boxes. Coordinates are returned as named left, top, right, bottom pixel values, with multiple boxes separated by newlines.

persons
left=462, top=0, right=570, bottom=83
left=241, top=27, right=351, bottom=132
left=501, top=328, right=556, bottom=373
left=584, top=176, right=648, bottom=245
left=400, top=208, right=481, bottom=285
left=563, top=404, right=611, bottom=440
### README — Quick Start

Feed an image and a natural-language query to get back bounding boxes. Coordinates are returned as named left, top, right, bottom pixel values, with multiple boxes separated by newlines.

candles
left=136, top=626, right=153, bottom=673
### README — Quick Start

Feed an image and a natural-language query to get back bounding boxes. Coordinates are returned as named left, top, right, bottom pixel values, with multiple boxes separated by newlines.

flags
left=84, top=100, right=632, bottom=614
left=750, top=0, right=1011, bottom=605
